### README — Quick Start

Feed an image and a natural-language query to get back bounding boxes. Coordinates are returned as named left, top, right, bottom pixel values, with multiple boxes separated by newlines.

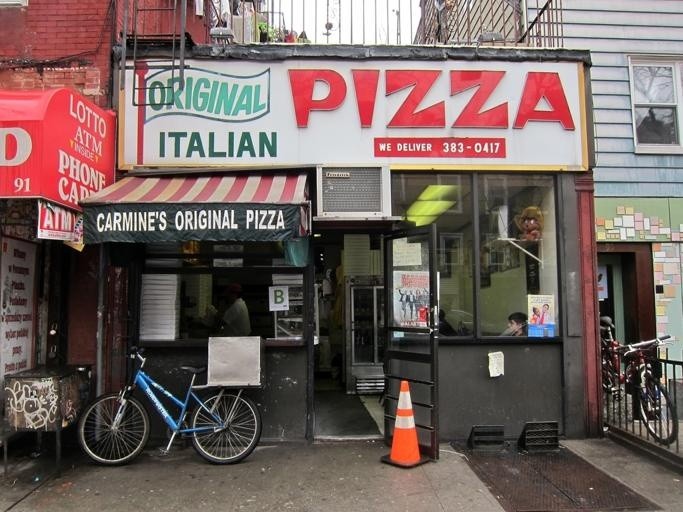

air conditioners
left=490, top=250, right=505, bottom=265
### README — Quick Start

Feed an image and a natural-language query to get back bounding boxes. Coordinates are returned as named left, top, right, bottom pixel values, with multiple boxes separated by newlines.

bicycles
left=599, top=314, right=680, bottom=448
left=75, top=344, right=264, bottom=468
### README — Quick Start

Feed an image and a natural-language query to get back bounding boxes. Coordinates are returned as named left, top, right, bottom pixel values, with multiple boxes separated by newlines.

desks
left=4, top=364, right=95, bottom=479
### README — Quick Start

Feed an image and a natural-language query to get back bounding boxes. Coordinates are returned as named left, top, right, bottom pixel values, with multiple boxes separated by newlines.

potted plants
left=258, top=23, right=267, bottom=41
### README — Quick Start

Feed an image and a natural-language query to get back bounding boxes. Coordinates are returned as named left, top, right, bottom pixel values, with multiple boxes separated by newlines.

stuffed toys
left=513, top=205, right=545, bottom=241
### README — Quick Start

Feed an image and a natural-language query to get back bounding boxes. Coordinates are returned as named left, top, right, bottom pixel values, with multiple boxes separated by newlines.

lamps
left=476, top=31, right=505, bottom=45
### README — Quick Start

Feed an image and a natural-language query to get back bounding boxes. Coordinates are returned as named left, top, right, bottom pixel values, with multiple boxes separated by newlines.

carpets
left=312, top=388, right=382, bottom=439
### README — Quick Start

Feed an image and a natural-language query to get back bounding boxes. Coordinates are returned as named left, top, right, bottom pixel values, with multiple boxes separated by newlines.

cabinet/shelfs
left=438, top=232, right=465, bottom=265
left=272, top=279, right=320, bottom=338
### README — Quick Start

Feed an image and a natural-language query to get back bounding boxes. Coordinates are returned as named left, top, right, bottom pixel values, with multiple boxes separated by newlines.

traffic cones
left=377, top=380, right=432, bottom=469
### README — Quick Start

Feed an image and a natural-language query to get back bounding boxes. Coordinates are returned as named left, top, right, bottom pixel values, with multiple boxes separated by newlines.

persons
left=208, top=282, right=251, bottom=336
left=500, top=312, right=527, bottom=336
left=530, top=304, right=549, bottom=325
left=439, top=309, right=458, bottom=336
left=399, top=289, right=429, bottom=319
